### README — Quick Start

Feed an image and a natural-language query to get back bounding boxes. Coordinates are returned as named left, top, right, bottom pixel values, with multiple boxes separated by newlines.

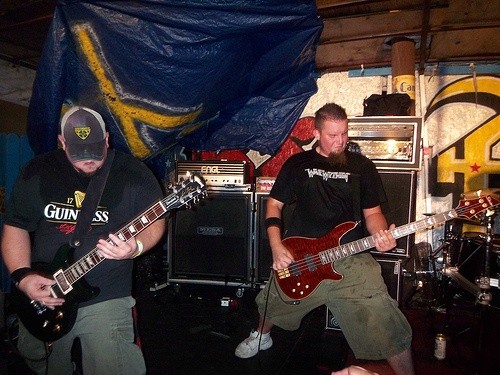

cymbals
left=422, top=212, right=483, bottom=226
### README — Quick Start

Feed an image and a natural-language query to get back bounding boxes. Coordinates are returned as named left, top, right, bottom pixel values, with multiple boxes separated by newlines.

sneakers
left=234, top=328, right=273, bottom=358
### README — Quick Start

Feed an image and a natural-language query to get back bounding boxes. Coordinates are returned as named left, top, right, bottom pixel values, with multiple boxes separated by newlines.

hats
left=61, top=107, right=107, bottom=163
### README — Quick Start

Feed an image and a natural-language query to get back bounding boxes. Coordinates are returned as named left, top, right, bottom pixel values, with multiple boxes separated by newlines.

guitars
left=272, top=195, right=500, bottom=299
left=10, top=170, right=209, bottom=342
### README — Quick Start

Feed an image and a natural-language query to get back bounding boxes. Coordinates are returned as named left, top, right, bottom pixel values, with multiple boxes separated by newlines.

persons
left=236, top=102, right=413, bottom=375
left=0, top=105, right=170, bottom=375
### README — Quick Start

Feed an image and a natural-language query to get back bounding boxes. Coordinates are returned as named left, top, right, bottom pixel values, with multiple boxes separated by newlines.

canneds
left=434, top=334, right=446, bottom=359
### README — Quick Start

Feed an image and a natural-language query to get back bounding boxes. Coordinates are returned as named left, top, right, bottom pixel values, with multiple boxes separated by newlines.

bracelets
left=11, top=267, right=34, bottom=288
left=265, top=217, right=282, bottom=230
left=136, top=240, right=143, bottom=256
